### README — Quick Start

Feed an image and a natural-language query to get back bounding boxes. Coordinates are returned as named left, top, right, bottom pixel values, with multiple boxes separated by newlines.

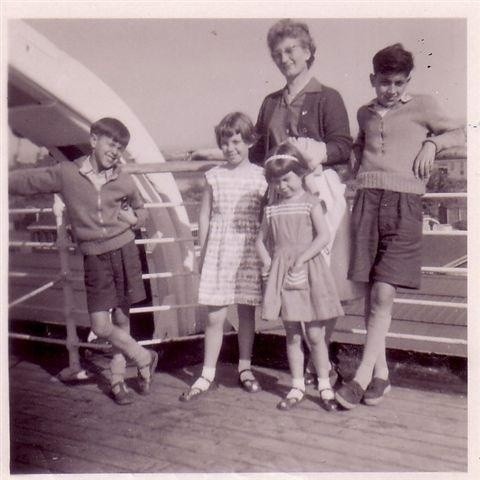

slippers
left=107, top=380, right=135, bottom=405
left=138, top=350, right=158, bottom=395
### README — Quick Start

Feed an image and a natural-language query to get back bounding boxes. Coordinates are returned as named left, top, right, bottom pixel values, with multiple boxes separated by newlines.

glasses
left=271, top=45, right=303, bottom=58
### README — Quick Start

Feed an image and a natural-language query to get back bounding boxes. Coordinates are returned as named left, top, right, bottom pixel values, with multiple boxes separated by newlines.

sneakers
left=335, top=378, right=364, bottom=408
left=363, top=377, right=392, bottom=405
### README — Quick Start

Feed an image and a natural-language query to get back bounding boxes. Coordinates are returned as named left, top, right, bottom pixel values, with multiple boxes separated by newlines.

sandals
left=320, top=389, right=339, bottom=411
left=238, top=368, right=261, bottom=393
left=180, top=375, right=218, bottom=402
left=279, top=385, right=306, bottom=408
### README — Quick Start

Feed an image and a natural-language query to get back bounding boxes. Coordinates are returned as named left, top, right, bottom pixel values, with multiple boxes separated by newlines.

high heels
left=304, top=371, right=317, bottom=385
left=330, top=360, right=345, bottom=387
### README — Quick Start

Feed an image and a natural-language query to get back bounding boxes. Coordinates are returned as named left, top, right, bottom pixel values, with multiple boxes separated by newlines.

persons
left=333, top=44, right=467, bottom=408
left=8, top=116, right=158, bottom=406
left=178, top=113, right=270, bottom=403
left=254, top=143, right=347, bottom=409
left=185, top=18, right=352, bottom=388
